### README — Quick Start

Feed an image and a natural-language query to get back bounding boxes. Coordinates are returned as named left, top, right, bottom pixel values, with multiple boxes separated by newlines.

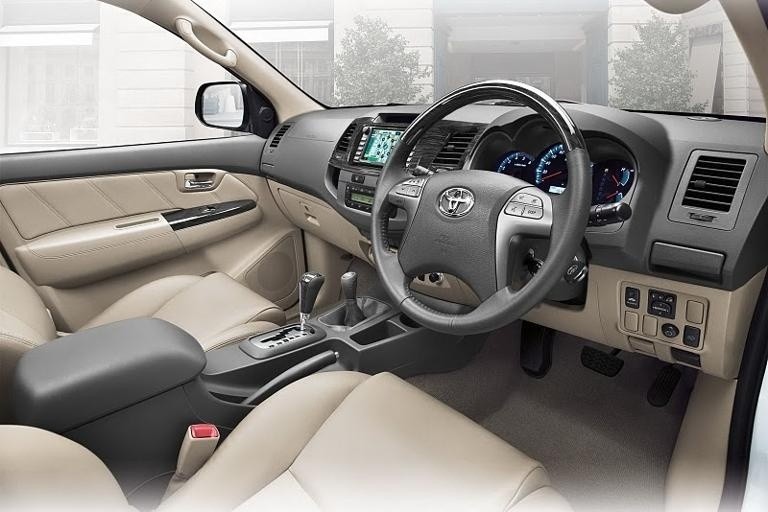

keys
left=526, top=254, right=545, bottom=278
left=566, top=259, right=590, bottom=289
